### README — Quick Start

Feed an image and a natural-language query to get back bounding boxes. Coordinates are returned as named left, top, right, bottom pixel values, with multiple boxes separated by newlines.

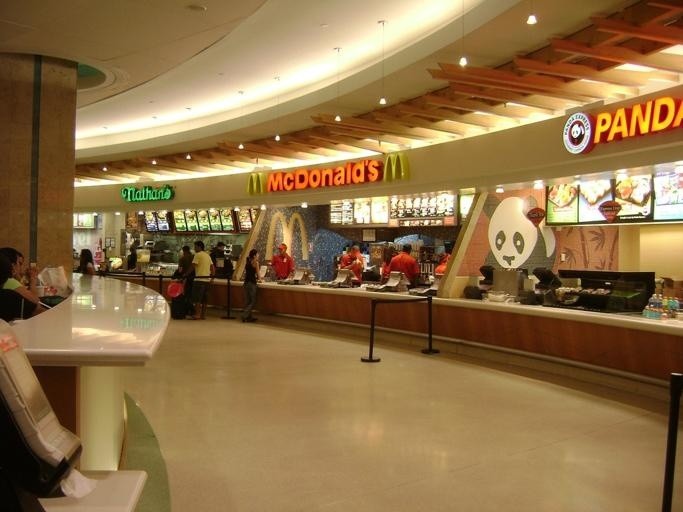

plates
left=548, top=183, right=578, bottom=207
left=580, top=183, right=611, bottom=206
left=615, top=181, right=652, bottom=206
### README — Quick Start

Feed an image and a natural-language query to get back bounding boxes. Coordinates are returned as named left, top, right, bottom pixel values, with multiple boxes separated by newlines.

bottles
left=643, top=293, right=679, bottom=320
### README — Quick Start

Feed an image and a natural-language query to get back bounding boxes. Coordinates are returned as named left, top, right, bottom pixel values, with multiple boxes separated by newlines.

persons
left=271, top=243, right=295, bottom=280
left=0, top=247, right=39, bottom=323
left=383, top=244, right=420, bottom=290
left=240, top=249, right=260, bottom=322
left=340, top=245, right=364, bottom=288
left=172, top=241, right=233, bottom=320
left=126, top=246, right=137, bottom=270
left=80, top=249, right=96, bottom=275
left=435, top=253, right=451, bottom=273
